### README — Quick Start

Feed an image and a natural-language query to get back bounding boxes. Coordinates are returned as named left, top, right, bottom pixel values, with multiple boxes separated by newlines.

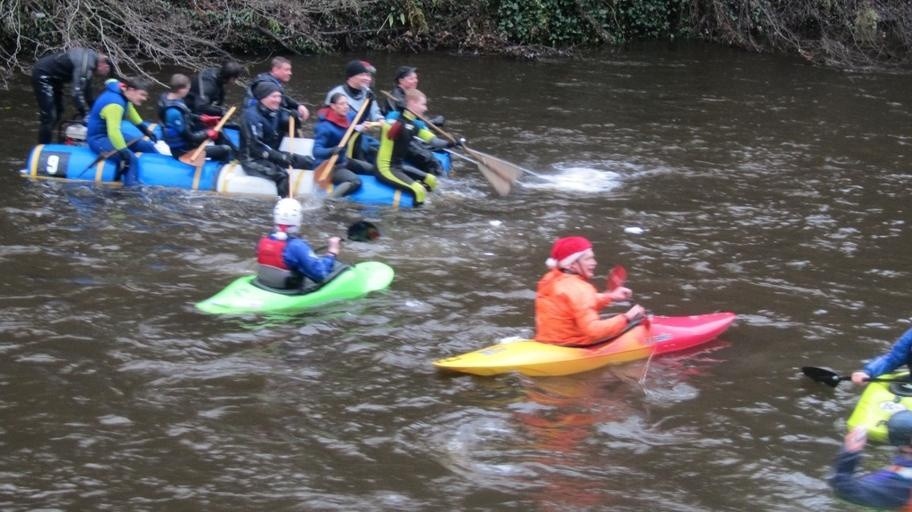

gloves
left=145, top=130, right=156, bottom=142
left=279, top=152, right=297, bottom=165
left=396, top=98, right=405, bottom=114
left=207, top=129, right=218, bottom=140
left=119, top=156, right=128, bottom=174
left=448, top=136, right=463, bottom=148
left=201, top=115, right=220, bottom=124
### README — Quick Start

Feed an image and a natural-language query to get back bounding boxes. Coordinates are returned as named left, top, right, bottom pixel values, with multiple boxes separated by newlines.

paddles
left=380, top=91, right=399, bottom=103
left=78, top=132, right=149, bottom=175
left=312, top=98, right=370, bottom=189
left=412, top=137, right=512, bottom=196
left=288, top=115, right=293, bottom=197
left=313, top=238, right=343, bottom=251
left=179, top=105, right=235, bottom=166
left=801, top=364, right=912, bottom=385
left=380, top=92, right=524, bottom=190
left=606, top=268, right=646, bottom=317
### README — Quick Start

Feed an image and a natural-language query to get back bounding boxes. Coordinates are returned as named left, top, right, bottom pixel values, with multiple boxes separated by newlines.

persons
left=324, top=61, right=387, bottom=135
left=852, top=331, right=908, bottom=386
left=157, top=73, right=232, bottom=163
left=31, top=45, right=112, bottom=145
left=257, top=199, right=342, bottom=288
left=243, top=58, right=311, bottom=139
left=313, top=94, right=373, bottom=198
left=381, top=66, right=467, bottom=148
left=374, top=87, right=463, bottom=208
left=831, top=410, right=912, bottom=511
left=87, top=76, right=160, bottom=188
left=534, top=236, right=644, bottom=348
left=239, top=81, right=314, bottom=200
left=189, top=62, right=242, bottom=129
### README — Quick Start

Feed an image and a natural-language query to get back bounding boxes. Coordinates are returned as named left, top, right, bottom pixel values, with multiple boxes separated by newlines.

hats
left=256, top=82, right=278, bottom=99
left=545, top=236, right=591, bottom=266
left=346, top=60, right=376, bottom=80
left=394, top=65, right=416, bottom=79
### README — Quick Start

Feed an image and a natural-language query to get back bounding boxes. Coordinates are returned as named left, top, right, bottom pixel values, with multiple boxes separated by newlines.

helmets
left=273, top=199, right=303, bottom=226
left=888, top=412, right=912, bottom=445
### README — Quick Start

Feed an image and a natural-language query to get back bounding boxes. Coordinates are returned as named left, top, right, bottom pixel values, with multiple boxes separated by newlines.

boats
left=433, top=306, right=736, bottom=377
left=846, top=368, right=908, bottom=449
left=22, top=142, right=430, bottom=211
left=63, top=118, right=454, bottom=182
left=195, top=257, right=396, bottom=320
